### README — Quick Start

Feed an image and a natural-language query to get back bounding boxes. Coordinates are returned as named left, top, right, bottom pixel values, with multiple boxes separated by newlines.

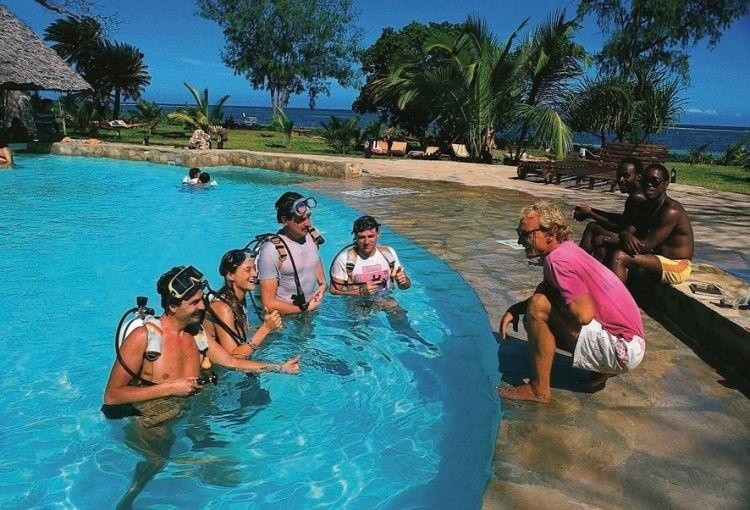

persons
left=34, top=98, right=57, bottom=143
left=197, top=172, right=219, bottom=186
left=103, top=264, right=301, bottom=510
left=591, top=163, right=695, bottom=285
left=182, top=167, right=201, bottom=184
left=573, top=158, right=646, bottom=257
left=329, top=215, right=438, bottom=351
left=0, top=142, right=13, bottom=167
left=257, top=192, right=328, bottom=315
left=496, top=199, right=646, bottom=403
left=200, top=249, right=282, bottom=360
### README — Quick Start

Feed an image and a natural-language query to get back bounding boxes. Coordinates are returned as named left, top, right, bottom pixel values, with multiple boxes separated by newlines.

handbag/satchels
left=603, top=237, right=610, bottom=249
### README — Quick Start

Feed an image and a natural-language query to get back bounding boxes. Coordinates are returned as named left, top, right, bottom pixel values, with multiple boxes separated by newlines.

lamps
left=452, top=143, right=471, bottom=160
left=425, top=146, right=441, bottom=160
left=371, top=140, right=389, bottom=158
left=387, top=141, right=407, bottom=158
left=517, top=142, right=668, bottom=192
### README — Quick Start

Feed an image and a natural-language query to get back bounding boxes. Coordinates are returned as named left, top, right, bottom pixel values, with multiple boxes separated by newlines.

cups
left=355, top=217, right=376, bottom=230
left=515, top=227, right=543, bottom=239
left=167, top=264, right=204, bottom=302
left=637, top=176, right=664, bottom=188
left=287, top=196, right=318, bottom=218
left=229, top=248, right=257, bottom=267
